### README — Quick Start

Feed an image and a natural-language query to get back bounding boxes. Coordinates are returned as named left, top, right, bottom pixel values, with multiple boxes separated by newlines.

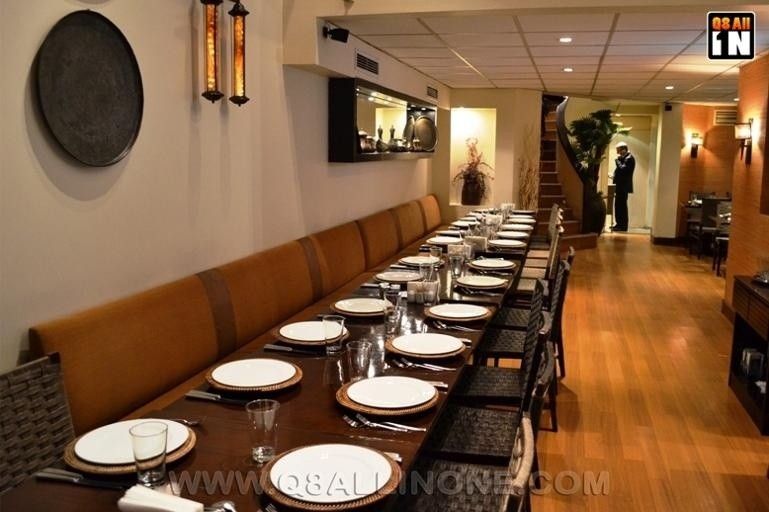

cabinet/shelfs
left=718, top=271, right=769, bottom=436
left=324, top=72, right=443, bottom=165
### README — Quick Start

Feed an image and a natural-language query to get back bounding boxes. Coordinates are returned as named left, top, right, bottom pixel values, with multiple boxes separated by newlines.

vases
left=458, top=177, right=483, bottom=206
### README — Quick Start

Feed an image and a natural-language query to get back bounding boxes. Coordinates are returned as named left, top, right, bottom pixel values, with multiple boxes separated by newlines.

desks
left=684, top=198, right=721, bottom=257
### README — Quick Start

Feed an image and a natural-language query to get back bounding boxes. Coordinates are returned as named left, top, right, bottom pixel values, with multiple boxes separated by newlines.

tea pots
left=358, top=124, right=423, bottom=153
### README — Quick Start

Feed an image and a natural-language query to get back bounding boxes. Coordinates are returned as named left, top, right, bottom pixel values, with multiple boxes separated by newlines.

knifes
left=35, top=465, right=129, bottom=493
left=185, top=390, right=246, bottom=409
left=264, top=344, right=318, bottom=357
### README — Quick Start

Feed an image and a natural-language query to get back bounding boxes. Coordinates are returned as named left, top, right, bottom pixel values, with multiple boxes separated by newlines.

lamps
left=728, top=107, right=762, bottom=171
left=222, top=0, right=251, bottom=105
left=684, top=127, right=710, bottom=168
left=318, top=22, right=348, bottom=46
left=194, top=0, right=226, bottom=104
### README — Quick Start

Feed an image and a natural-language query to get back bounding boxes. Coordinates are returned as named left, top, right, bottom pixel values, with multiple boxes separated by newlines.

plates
left=392, top=214, right=535, bottom=354
left=338, top=212, right=481, bottom=313
left=350, top=375, right=437, bottom=410
left=75, top=418, right=191, bottom=465
left=280, top=321, right=348, bottom=341
left=267, top=444, right=393, bottom=505
left=211, top=357, right=296, bottom=389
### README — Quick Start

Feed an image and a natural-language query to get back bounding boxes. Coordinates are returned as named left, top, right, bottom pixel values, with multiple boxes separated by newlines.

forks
left=340, top=413, right=427, bottom=436
left=475, top=267, right=513, bottom=277
left=265, top=503, right=278, bottom=511
left=432, top=321, right=484, bottom=335
left=460, top=285, right=503, bottom=300
left=394, top=357, right=459, bottom=374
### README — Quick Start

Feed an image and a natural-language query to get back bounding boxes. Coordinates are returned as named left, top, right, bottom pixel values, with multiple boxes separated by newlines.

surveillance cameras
left=327, top=28, right=349, bottom=43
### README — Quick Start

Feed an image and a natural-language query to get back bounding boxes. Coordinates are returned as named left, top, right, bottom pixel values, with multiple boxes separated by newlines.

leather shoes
left=609, top=226, right=628, bottom=231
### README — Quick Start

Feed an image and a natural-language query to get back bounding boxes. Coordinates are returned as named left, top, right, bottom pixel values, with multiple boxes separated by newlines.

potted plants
left=572, top=107, right=633, bottom=238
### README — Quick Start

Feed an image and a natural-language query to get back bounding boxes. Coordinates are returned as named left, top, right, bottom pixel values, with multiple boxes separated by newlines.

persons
left=608, top=144, right=633, bottom=232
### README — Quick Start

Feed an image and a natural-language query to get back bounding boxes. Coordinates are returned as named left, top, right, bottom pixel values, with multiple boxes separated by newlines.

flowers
left=451, top=134, right=496, bottom=195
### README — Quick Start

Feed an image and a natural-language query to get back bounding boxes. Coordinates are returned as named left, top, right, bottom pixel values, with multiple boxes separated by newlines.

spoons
left=211, top=499, right=239, bottom=512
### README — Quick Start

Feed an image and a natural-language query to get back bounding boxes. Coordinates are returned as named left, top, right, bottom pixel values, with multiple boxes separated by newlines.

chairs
left=713, top=200, right=729, bottom=281
left=683, top=191, right=713, bottom=238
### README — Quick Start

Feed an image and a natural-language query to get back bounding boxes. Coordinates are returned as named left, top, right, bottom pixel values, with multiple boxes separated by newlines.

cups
left=344, top=339, right=373, bottom=378
left=741, top=348, right=764, bottom=381
left=127, top=421, right=168, bottom=487
left=382, top=206, right=510, bottom=336
left=690, top=191, right=698, bottom=206
left=322, top=314, right=346, bottom=355
left=245, top=396, right=283, bottom=465
